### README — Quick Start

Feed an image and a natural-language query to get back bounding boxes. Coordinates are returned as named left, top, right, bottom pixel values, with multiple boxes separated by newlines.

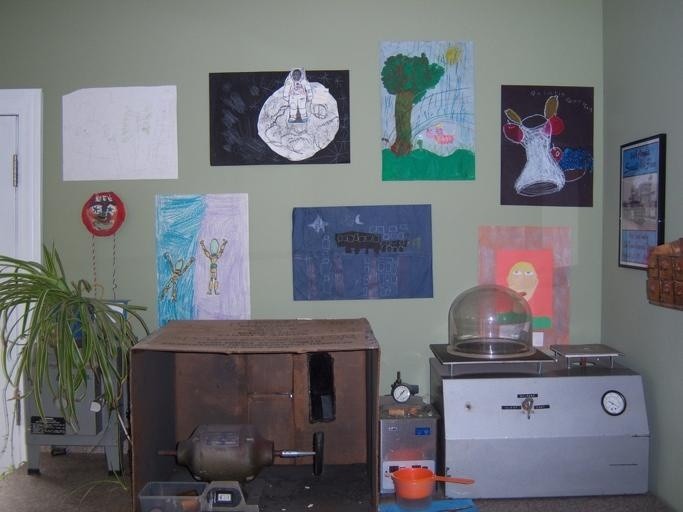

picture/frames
left=618, top=131, right=668, bottom=270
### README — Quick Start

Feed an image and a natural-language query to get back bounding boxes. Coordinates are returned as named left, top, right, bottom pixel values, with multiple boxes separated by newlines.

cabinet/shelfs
left=379, top=395, right=440, bottom=499
left=127, top=319, right=382, bottom=512
left=25, top=341, right=131, bottom=477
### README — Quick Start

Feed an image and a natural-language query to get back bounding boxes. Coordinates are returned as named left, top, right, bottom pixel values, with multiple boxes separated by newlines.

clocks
left=392, top=384, right=411, bottom=403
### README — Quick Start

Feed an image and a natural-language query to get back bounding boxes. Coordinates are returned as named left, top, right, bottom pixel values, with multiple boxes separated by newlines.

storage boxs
left=137, top=481, right=208, bottom=512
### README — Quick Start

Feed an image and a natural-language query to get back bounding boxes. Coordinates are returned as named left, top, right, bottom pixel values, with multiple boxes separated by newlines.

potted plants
left=0, top=242, right=148, bottom=448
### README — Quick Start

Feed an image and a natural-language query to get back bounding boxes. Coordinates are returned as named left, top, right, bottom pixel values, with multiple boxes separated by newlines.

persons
left=281, top=68, right=313, bottom=124
